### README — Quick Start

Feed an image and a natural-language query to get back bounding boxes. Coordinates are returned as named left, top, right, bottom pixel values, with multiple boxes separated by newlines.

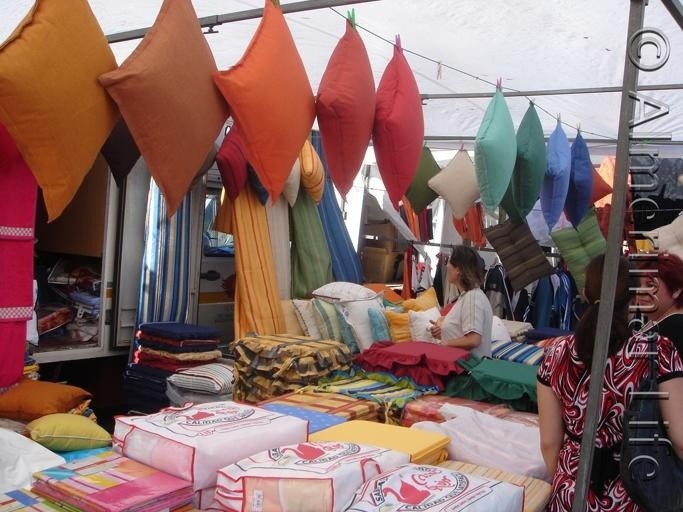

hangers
left=403, top=240, right=504, bottom=275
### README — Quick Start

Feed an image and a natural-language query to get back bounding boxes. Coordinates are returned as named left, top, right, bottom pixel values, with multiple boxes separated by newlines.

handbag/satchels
left=620, top=330, right=683, bottom=511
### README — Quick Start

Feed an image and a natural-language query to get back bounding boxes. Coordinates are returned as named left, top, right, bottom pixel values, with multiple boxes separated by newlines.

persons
left=536, top=252, right=683, bottom=512
left=430, top=246, right=493, bottom=359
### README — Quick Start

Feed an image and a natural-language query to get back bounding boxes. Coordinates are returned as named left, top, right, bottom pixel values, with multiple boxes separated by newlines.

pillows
left=281, top=281, right=441, bottom=354
left=1, top=377, right=113, bottom=453
left=2, top=0, right=614, bottom=229
